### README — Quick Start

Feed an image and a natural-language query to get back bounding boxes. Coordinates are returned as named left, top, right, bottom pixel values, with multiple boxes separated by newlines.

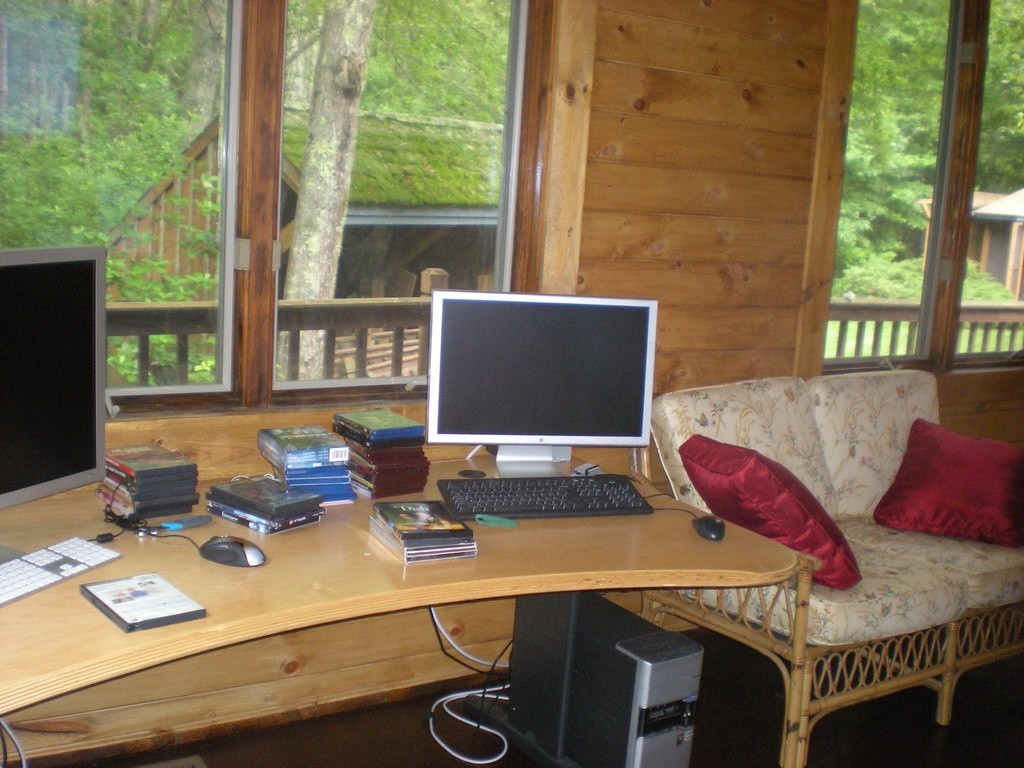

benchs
left=640, top=368, right=1024, bottom=768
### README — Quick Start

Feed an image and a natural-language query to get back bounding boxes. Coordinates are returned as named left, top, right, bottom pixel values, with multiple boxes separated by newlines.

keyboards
left=436, top=474, right=654, bottom=522
left=0, top=536, right=122, bottom=606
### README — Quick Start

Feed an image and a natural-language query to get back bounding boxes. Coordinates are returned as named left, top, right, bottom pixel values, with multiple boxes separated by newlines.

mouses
left=692, top=515, right=725, bottom=541
left=197, top=535, right=267, bottom=567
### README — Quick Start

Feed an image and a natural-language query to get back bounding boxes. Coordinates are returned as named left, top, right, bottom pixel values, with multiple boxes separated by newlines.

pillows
left=873, top=419, right=1024, bottom=548
left=678, top=433, right=863, bottom=590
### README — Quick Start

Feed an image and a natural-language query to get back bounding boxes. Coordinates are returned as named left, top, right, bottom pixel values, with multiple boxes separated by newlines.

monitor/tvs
left=0, top=245, right=108, bottom=565
left=425, top=288, right=659, bottom=478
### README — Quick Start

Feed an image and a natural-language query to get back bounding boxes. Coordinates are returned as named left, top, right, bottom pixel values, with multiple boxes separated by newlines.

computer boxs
left=503, top=592, right=705, bottom=768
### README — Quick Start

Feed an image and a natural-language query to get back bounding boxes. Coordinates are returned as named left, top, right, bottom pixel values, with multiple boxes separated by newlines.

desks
left=0, top=455, right=805, bottom=768
